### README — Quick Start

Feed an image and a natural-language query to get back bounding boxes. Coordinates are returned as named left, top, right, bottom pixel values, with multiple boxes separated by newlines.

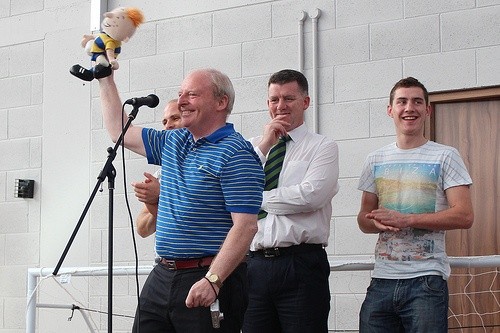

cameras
left=210, top=299, right=224, bottom=328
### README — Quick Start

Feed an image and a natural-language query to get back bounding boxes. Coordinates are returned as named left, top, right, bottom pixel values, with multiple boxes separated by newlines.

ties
left=257, top=133, right=291, bottom=220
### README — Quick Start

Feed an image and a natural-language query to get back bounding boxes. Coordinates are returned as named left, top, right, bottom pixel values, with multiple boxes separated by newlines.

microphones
left=125, top=94, right=160, bottom=108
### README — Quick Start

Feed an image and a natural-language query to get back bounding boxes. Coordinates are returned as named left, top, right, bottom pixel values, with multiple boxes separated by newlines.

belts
left=250, top=244, right=322, bottom=258
left=159, top=255, right=214, bottom=270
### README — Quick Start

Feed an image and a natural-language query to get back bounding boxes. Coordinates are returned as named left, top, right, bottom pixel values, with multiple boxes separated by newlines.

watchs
left=204, top=271, right=223, bottom=289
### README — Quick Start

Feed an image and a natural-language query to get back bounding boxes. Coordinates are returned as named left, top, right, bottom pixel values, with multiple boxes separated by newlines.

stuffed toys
left=70, top=7, right=144, bottom=81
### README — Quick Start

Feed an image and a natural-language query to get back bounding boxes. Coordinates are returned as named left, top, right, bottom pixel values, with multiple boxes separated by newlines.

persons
left=242, top=68, right=340, bottom=333
left=356, top=77, right=474, bottom=333
left=81, top=34, right=266, bottom=333
